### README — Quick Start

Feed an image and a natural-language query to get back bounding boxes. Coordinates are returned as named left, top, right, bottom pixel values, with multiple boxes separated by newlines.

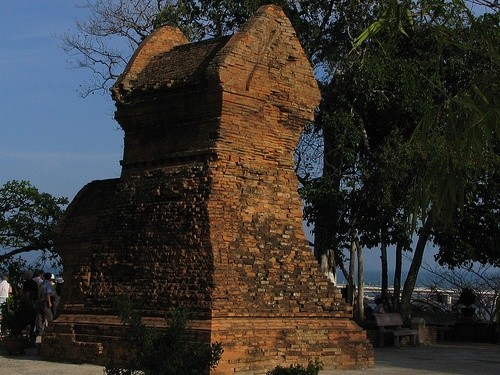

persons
left=0, top=268, right=64, bottom=356
